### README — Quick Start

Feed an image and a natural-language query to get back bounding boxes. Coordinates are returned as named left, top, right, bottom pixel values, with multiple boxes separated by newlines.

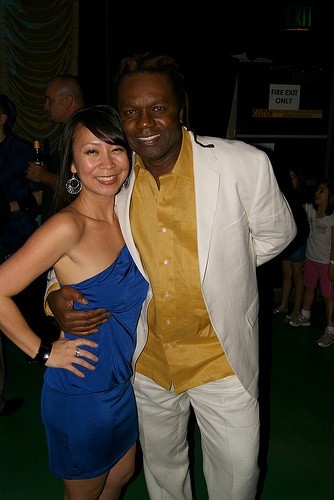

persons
left=44, top=53, right=298, bottom=500
left=25, top=74, right=88, bottom=226
left=0, top=94, right=43, bottom=266
left=289, top=180, right=334, bottom=347
left=272, top=169, right=310, bottom=324
left=0, top=104, right=149, bottom=500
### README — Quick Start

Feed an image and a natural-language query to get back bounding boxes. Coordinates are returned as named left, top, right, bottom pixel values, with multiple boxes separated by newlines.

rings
left=76, top=348, right=80, bottom=356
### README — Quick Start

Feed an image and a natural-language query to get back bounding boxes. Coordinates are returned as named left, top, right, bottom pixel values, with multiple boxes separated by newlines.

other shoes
left=281, top=312, right=301, bottom=323
left=316, top=328, right=334, bottom=347
left=272, top=307, right=288, bottom=316
left=289, top=314, right=311, bottom=327
left=0, top=397, right=24, bottom=416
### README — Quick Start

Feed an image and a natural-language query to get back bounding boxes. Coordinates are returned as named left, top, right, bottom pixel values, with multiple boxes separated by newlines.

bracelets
left=34, top=340, right=52, bottom=365
left=330, top=261, right=334, bottom=264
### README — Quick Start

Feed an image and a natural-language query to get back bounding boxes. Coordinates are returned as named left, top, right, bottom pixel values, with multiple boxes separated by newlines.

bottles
left=32, top=136, right=45, bottom=169
